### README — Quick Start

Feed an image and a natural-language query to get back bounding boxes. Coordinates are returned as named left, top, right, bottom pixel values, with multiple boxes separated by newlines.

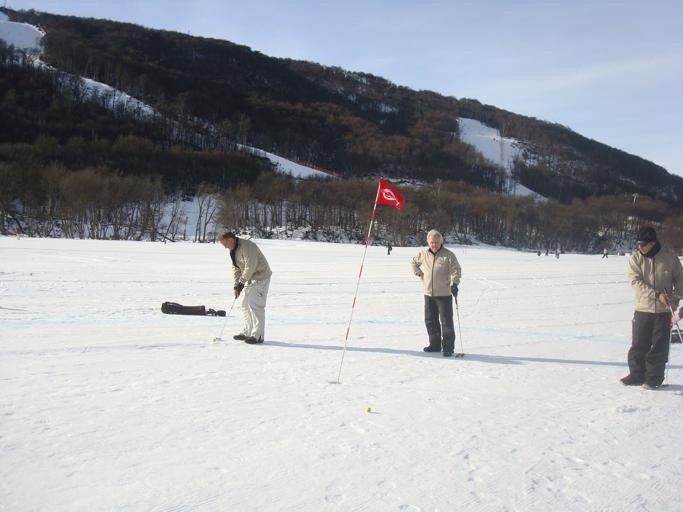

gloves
left=450, top=284, right=459, bottom=296
left=234, top=283, right=245, bottom=299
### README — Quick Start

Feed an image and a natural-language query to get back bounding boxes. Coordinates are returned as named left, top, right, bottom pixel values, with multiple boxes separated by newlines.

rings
left=675, top=322, right=676, bottom=324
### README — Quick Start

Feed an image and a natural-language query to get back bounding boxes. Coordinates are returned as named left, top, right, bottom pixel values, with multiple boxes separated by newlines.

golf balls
left=366, top=407, right=371, bottom=413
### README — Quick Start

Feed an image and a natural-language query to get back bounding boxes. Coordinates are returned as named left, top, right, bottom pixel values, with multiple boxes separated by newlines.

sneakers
left=246, top=336, right=265, bottom=344
left=642, top=380, right=664, bottom=389
left=620, top=374, right=645, bottom=386
left=424, top=344, right=441, bottom=353
left=443, top=349, right=455, bottom=356
left=234, top=334, right=246, bottom=339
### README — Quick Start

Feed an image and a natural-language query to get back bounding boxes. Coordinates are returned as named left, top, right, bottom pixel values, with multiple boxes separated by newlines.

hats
left=636, top=226, right=657, bottom=242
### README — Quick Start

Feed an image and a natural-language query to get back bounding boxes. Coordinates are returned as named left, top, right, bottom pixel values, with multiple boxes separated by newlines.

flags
left=376, top=178, right=403, bottom=210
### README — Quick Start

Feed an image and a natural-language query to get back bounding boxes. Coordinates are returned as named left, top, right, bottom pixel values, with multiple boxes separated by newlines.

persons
left=671, top=307, right=682, bottom=326
left=601, top=246, right=608, bottom=259
left=556, top=248, right=559, bottom=259
left=387, top=241, right=392, bottom=255
left=411, top=228, right=462, bottom=356
left=217, top=230, right=272, bottom=343
left=618, top=225, right=682, bottom=389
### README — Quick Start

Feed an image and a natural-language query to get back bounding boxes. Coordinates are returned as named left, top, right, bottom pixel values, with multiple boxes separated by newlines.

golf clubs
left=212, top=296, right=237, bottom=341
left=455, top=297, right=465, bottom=358
